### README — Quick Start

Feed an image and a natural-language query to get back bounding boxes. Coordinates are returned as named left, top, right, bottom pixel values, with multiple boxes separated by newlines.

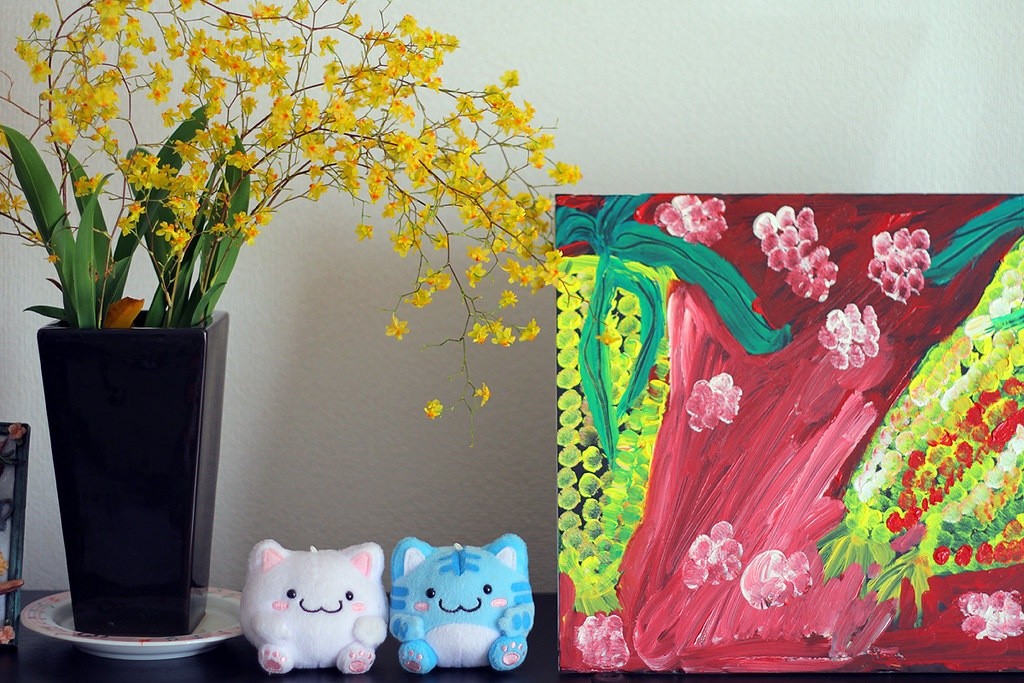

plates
left=18, top=588, right=257, bottom=661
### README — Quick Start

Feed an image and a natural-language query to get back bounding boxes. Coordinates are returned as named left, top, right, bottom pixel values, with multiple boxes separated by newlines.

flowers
left=0, top=0, right=625, bottom=451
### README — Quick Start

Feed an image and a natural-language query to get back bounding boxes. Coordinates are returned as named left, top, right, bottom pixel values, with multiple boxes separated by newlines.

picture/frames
left=0, top=420, right=37, bottom=651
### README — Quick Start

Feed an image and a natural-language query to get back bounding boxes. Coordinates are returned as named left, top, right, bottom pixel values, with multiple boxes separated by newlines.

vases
left=31, top=312, right=232, bottom=637
